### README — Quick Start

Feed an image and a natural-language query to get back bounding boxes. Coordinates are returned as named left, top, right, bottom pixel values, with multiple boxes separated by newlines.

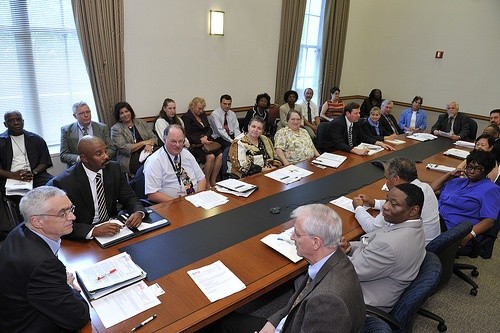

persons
left=143, top=124, right=207, bottom=203
left=0, top=186, right=90, bottom=333
left=353, top=158, right=440, bottom=248
left=60, top=101, right=117, bottom=169
left=397, top=95, right=427, bottom=133
left=360, top=87, right=404, bottom=141
left=429, top=149, right=500, bottom=254
left=154, top=94, right=243, bottom=188
left=196, top=204, right=366, bottom=333
left=294, top=183, right=427, bottom=318
left=274, top=109, right=320, bottom=166
left=431, top=101, right=473, bottom=142
left=244, top=87, right=346, bottom=142
left=111, top=101, right=160, bottom=175
left=0, top=109, right=55, bottom=223
left=229, top=117, right=283, bottom=180
left=320, top=102, right=395, bottom=156
left=456, top=109, right=500, bottom=180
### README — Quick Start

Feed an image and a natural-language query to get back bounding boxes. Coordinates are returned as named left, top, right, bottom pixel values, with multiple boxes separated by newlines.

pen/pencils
left=279, top=175, right=290, bottom=180
left=363, top=146, right=367, bottom=150
left=277, top=238, right=294, bottom=244
left=130, top=313, right=157, bottom=332
left=98, top=268, right=116, bottom=280
left=311, top=158, right=322, bottom=163
left=234, top=184, right=245, bottom=190
left=435, top=162, right=440, bottom=168
left=420, top=179, right=433, bottom=184
left=429, top=162, right=433, bottom=168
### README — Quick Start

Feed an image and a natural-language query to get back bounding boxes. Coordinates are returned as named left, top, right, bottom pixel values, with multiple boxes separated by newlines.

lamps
left=208, top=9, right=225, bottom=36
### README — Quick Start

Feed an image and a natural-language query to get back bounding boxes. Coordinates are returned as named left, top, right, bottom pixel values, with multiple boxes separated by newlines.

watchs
left=52, top=135, right=150, bottom=243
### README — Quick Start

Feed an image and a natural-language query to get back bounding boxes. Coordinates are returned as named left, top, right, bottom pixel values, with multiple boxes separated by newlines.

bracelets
left=471, top=230, right=477, bottom=238
left=136, top=211, right=145, bottom=219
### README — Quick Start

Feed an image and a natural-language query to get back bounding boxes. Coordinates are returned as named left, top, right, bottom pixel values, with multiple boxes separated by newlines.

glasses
left=75, top=110, right=91, bottom=116
left=290, top=230, right=313, bottom=240
left=466, top=165, right=485, bottom=172
left=36, top=205, right=76, bottom=218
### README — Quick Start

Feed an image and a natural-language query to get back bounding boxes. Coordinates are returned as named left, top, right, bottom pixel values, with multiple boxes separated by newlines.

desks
left=57, top=129, right=484, bottom=333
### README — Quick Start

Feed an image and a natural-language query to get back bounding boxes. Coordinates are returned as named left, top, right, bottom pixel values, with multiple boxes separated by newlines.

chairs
left=360, top=175, right=500, bottom=333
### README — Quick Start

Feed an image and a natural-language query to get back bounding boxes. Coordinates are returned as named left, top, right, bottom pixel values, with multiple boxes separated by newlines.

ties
left=82, top=127, right=88, bottom=136
left=307, top=103, right=312, bottom=124
left=224, top=113, right=229, bottom=135
left=174, top=156, right=195, bottom=196
left=385, top=116, right=399, bottom=135
left=445, top=117, right=454, bottom=133
left=95, top=173, right=109, bottom=222
left=288, top=272, right=312, bottom=314
left=348, top=124, right=353, bottom=147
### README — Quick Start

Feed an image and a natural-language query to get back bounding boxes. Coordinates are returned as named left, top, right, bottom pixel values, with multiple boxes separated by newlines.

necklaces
left=77, top=124, right=89, bottom=136
left=10, top=136, right=31, bottom=172
left=129, top=126, right=137, bottom=144
left=162, top=145, right=185, bottom=199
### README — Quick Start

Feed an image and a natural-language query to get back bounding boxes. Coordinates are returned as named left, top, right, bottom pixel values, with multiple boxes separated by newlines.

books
left=312, top=151, right=348, bottom=169
left=264, top=164, right=314, bottom=185
left=260, top=226, right=304, bottom=264
left=438, top=131, right=453, bottom=138
left=384, top=139, right=407, bottom=145
left=407, top=132, right=438, bottom=142
left=91, top=207, right=171, bottom=249
left=443, top=148, right=470, bottom=160
